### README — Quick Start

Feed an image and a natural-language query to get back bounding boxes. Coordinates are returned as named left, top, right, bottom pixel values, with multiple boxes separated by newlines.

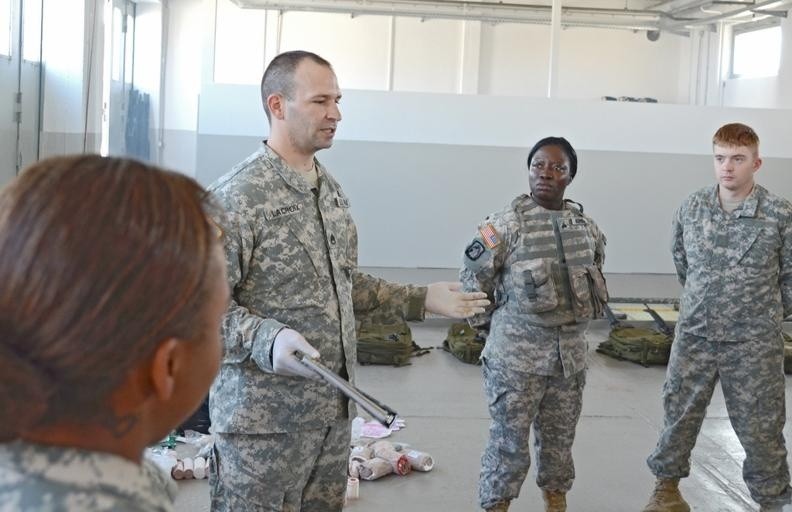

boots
left=644, top=477, right=690, bottom=512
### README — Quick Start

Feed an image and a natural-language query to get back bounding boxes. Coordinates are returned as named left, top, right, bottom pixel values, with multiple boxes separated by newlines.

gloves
left=272, top=328, right=320, bottom=378
left=425, top=282, right=490, bottom=318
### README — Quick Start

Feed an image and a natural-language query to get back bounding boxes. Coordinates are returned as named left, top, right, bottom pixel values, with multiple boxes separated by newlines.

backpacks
left=357, top=324, right=422, bottom=367
left=437, top=322, right=484, bottom=365
left=596, top=325, right=674, bottom=367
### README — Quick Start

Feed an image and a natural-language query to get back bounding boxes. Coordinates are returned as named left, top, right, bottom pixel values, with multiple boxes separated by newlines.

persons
left=642, top=123, right=791, bottom=512
left=458, top=136, right=610, bottom=512
left=0, top=154, right=230, bottom=512
left=197, top=50, right=491, bottom=512
